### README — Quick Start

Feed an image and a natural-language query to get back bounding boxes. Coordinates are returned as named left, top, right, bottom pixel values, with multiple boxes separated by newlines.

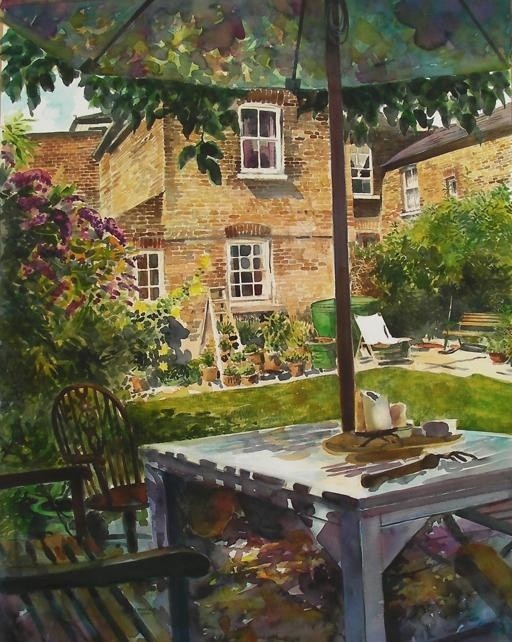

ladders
left=197, top=285, right=243, bottom=384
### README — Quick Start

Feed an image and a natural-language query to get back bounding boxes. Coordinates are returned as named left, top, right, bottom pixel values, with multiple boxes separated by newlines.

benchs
left=442, top=499, right=512, bottom=559
left=0, top=463, right=210, bottom=640
left=441, top=312, right=502, bottom=350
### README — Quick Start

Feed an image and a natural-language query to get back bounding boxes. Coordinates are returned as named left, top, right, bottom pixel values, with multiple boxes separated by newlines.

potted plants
left=480, top=333, right=508, bottom=363
left=200, top=309, right=320, bottom=386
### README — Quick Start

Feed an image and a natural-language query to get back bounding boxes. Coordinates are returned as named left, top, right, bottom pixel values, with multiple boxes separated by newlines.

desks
left=137, top=417, right=512, bottom=642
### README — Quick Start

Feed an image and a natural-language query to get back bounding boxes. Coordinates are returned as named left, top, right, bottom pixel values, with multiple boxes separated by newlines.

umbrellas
left=0, top=1, right=511, bottom=438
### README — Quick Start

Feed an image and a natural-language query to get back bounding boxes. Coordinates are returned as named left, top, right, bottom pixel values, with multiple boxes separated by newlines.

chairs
left=354, top=310, right=414, bottom=367
left=51, top=382, right=211, bottom=555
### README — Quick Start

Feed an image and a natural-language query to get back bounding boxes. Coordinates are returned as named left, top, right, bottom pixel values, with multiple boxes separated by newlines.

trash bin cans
left=310, top=297, right=337, bottom=339
left=350, top=295, right=380, bottom=356
